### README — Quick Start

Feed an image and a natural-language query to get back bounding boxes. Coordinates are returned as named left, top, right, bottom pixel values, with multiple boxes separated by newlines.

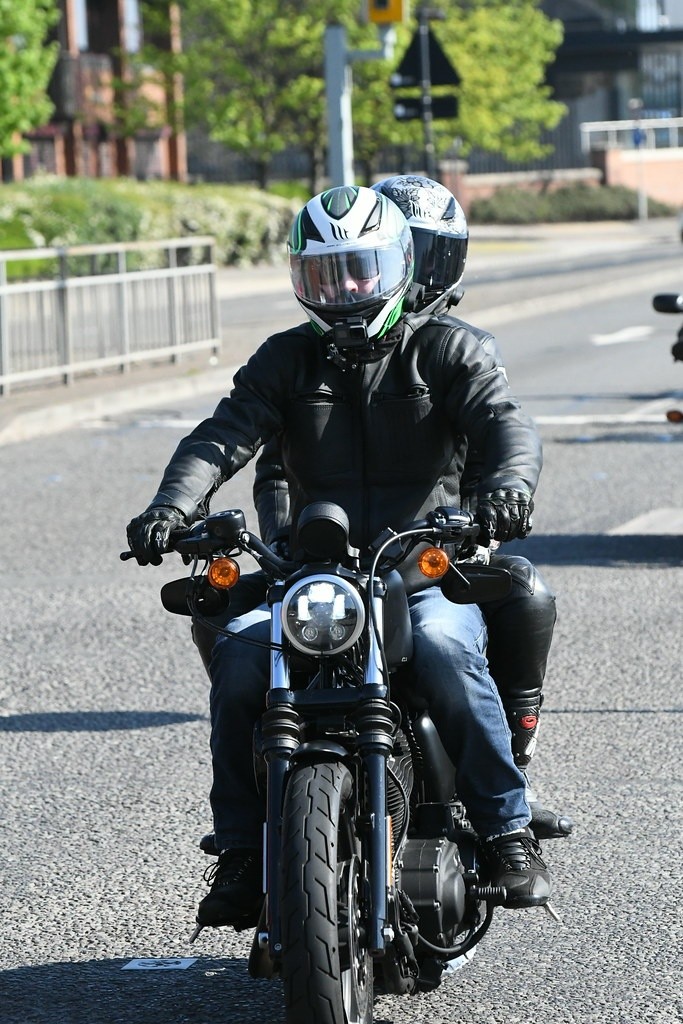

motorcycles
left=116, top=500, right=534, bottom=1024
left=651, top=292, right=683, bottom=427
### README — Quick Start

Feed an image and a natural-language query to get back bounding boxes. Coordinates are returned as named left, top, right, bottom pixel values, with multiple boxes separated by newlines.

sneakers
left=481, top=824, right=553, bottom=909
left=199, top=847, right=263, bottom=927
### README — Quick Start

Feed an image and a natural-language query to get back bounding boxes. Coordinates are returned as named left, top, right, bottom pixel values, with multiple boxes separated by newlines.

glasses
left=308, top=256, right=377, bottom=285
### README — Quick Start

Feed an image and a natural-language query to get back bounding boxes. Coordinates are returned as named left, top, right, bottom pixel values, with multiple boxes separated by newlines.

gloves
left=477, top=488, right=531, bottom=547
left=127, top=505, right=193, bottom=566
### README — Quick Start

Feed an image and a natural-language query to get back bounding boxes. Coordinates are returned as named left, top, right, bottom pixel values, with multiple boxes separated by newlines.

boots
left=497, top=678, right=573, bottom=838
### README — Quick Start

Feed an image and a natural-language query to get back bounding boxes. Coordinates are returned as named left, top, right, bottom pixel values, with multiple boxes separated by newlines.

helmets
left=290, top=186, right=414, bottom=348
left=371, top=174, right=468, bottom=311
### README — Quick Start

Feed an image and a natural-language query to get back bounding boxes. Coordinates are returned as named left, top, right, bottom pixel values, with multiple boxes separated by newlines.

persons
left=127, top=173, right=572, bottom=926
